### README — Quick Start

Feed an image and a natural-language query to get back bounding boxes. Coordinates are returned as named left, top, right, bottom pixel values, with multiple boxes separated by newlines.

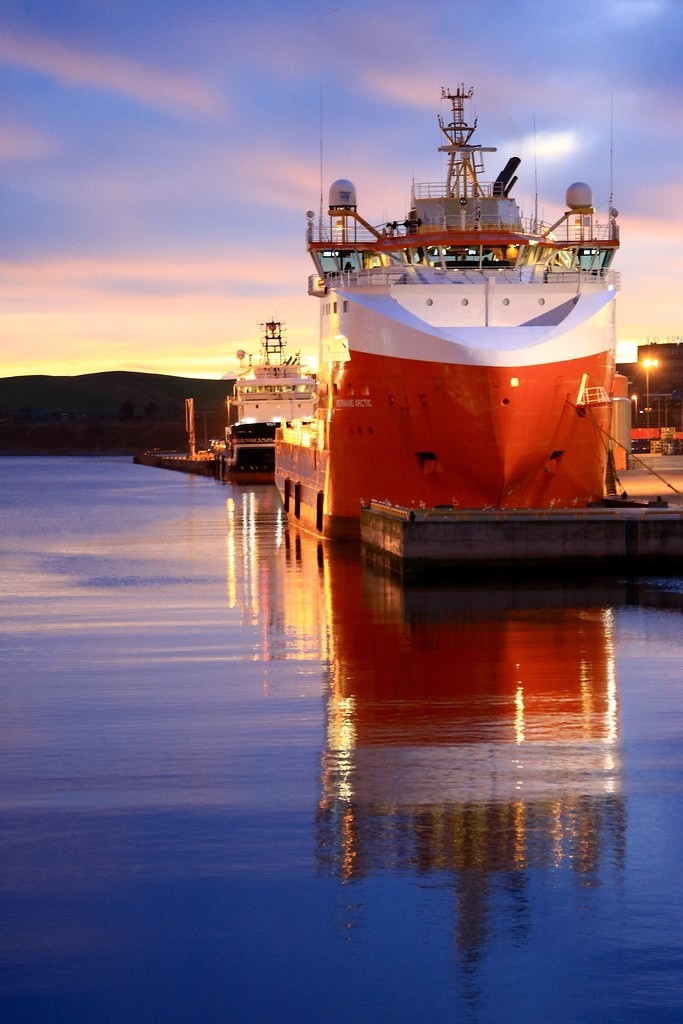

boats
left=217, top=319, right=321, bottom=485
left=274, top=81, right=622, bottom=537
